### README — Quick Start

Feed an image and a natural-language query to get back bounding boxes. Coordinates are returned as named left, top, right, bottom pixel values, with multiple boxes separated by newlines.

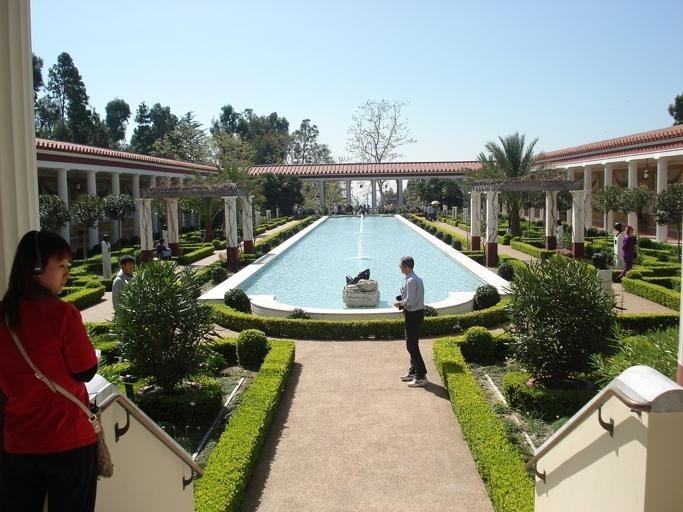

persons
left=613, top=223, right=625, bottom=267
left=157, top=239, right=171, bottom=259
left=112, top=255, right=136, bottom=309
left=394, top=256, right=429, bottom=387
left=0, top=231, right=98, bottom=512
left=293, top=204, right=304, bottom=220
left=424, top=203, right=440, bottom=222
left=162, top=225, right=170, bottom=249
left=555, top=218, right=563, bottom=249
left=332, top=202, right=396, bottom=214
left=101, top=234, right=112, bottom=277
left=616, top=226, right=637, bottom=282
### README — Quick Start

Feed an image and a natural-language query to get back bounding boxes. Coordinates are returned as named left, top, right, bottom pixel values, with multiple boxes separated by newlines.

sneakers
left=407, top=376, right=428, bottom=387
left=400, top=372, right=416, bottom=381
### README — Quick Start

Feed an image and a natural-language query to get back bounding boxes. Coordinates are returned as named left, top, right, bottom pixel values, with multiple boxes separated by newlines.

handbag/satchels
left=88, top=401, right=114, bottom=477
